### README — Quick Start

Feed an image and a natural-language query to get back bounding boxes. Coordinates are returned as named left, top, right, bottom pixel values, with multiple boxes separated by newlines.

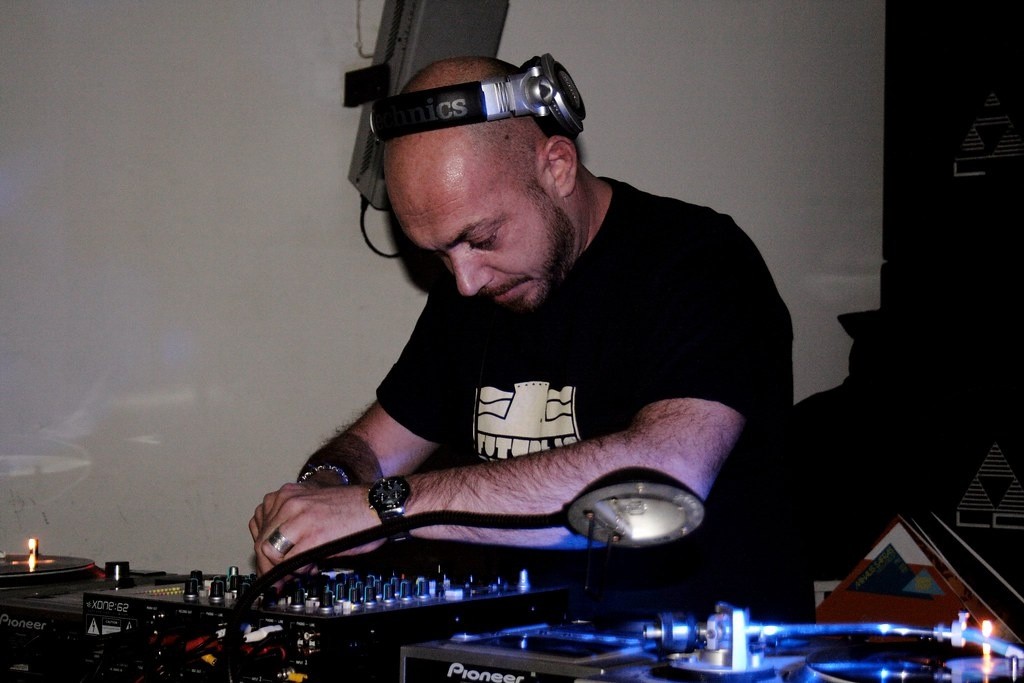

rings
left=268, top=530, right=294, bottom=554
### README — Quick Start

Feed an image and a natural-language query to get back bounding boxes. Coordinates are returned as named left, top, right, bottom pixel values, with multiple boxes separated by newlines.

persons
left=248, top=55, right=795, bottom=585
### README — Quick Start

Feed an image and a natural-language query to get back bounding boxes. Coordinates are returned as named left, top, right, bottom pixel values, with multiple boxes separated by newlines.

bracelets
left=299, top=465, right=348, bottom=485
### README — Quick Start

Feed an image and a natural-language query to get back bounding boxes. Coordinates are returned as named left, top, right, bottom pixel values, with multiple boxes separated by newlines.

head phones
left=369, top=54, right=586, bottom=142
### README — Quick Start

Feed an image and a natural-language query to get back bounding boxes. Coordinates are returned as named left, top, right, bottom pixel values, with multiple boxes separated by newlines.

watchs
left=368, top=476, right=411, bottom=541
